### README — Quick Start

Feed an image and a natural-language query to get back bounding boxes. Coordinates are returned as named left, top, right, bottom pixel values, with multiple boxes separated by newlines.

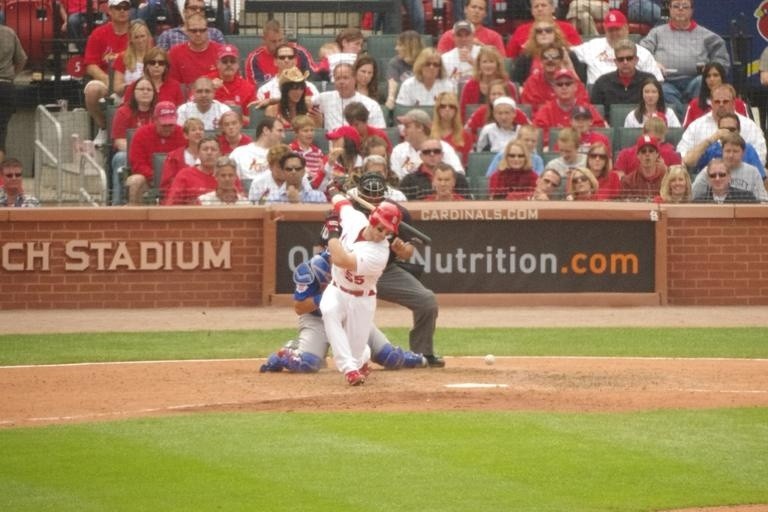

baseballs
left=485, top=354, right=495, bottom=365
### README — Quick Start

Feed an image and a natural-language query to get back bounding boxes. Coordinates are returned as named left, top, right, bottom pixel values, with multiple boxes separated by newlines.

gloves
left=319, top=217, right=339, bottom=246
left=323, top=179, right=339, bottom=202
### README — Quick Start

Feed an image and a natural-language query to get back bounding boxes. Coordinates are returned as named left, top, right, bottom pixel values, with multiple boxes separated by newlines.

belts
left=332, top=279, right=376, bottom=297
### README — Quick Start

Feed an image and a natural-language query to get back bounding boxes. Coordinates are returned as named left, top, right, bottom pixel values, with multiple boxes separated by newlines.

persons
left=582, top=142, right=621, bottom=201
left=123, top=46, right=186, bottom=108
left=652, top=164, right=693, bottom=203
left=160, top=1, right=228, bottom=48
left=228, top=119, right=287, bottom=178
left=488, top=143, right=539, bottom=201
left=319, top=182, right=402, bottom=386
left=620, top=135, right=670, bottom=200
left=167, top=13, right=235, bottom=87
left=543, top=128, right=590, bottom=174
left=514, top=17, right=587, bottom=85
left=321, top=125, right=367, bottom=185
left=532, top=69, right=604, bottom=128
left=421, top=164, right=465, bottom=201
left=521, top=45, right=588, bottom=105
left=485, top=125, right=545, bottom=176
left=262, top=152, right=327, bottom=204
left=399, top=140, right=468, bottom=200
left=346, top=154, right=406, bottom=202
left=590, top=38, right=663, bottom=104
left=462, top=80, right=535, bottom=154
left=586, top=10, right=664, bottom=83
left=110, top=22, right=160, bottom=97
left=683, top=114, right=767, bottom=182
left=438, top=22, right=494, bottom=86
left=351, top=54, right=383, bottom=102
left=82, top=0, right=157, bottom=147
left=320, top=30, right=365, bottom=79
left=110, top=77, right=161, bottom=205
left=459, top=45, right=519, bottom=107
left=176, top=77, right=235, bottom=128
left=192, top=43, right=259, bottom=120
left=245, top=17, right=321, bottom=83
left=389, top=109, right=464, bottom=181
left=553, top=106, right=613, bottom=170
left=475, top=97, right=526, bottom=154
left=514, top=0, right=582, bottom=53
left=503, top=167, right=563, bottom=201
left=195, top=156, right=254, bottom=207
left=321, top=173, right=446, bottom=367
left=255, top=42, right=318, bottom=101
left=1, top=21, right=29, bottom=154
left=385, top=29, right=437, bottom=109
left=159, top=117, right=216, bottom=204
left=382, top=0, right=426, bottom=35
left=0, top=159, right=40, bottom=208
left=396, top=47, right=454, bottom=107
left=625, top=77, right=680, bottom=127
left=357, top=135, right=400, bottom=186
left=285, top=114, right=327, bottom=181
left=567, top=168, right=606, bottom=200
left=340, top=101, right=392, bottom=152
left=689, top=133, right=768, bottom=201
left=637, top=0, right=730, bottom=117
left=247, top=145, right=313, bottom=200
left=261, top=244, right=428, bottom=379
left=682, top=60, right=749, bottom=126
left=430, top=92, right=475, bottom=152
left=168, top=140, right=246, bottom=208
left=674, top=84, right=767, bottom=168
left=615, top=116, right=681, bottom=166
left=437, top=0, right=510, bottom=58
left=265, top=65, right=323, bottom=127
left=125, top=101, right=190, bottom=206
left=215, top=111, right=255, bottom=153
left=693, top=158, right=756, bottom=203
left=313, top=63, right=387, bottom=133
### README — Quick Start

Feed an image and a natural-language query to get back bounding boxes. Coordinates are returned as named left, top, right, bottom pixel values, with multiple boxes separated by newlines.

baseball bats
left=329, top=179, right=432, bottom=245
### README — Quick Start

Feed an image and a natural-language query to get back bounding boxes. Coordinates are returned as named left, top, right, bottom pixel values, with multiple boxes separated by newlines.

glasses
left=5, top=173, right=22, bottom=178
left=108, top=1, right=739, bottom=185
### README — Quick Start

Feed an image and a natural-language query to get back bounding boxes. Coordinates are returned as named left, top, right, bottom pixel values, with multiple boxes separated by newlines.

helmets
left=368, top=201, right=402, bottom=236
left=358, top=171, right=386, bottom=206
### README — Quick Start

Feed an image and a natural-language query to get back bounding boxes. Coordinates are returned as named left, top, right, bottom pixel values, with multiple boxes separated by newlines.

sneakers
left=359, top=363, right=372, bottom=376
left=403, top=351, right=427, bottom=369
left=347, top=371, right=366, bottom=386
left=259, top=346, right=289, bottom=373
left=421, top=354, right=444, bottom=368
left=93, top=127, right=109, bottom=148
